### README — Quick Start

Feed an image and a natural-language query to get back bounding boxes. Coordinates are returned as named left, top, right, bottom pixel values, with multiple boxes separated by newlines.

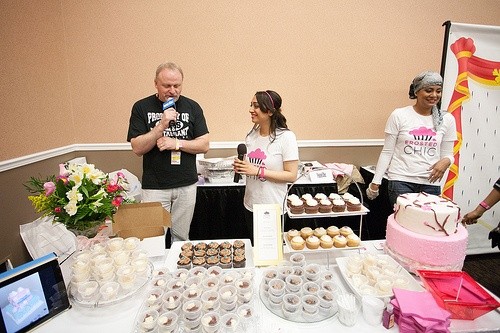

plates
left=259, top=276, right=342, bottom=323
left=164, top=239, right=256, bottom=271
left=66, top=261, right=154, bottom=307
left=334, top=253, right=426, bottom=300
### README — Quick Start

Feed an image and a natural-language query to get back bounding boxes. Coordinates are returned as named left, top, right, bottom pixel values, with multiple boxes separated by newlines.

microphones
left=163, top=97, right=176, bottom=128
left=234, top=144, right=247, bottom=183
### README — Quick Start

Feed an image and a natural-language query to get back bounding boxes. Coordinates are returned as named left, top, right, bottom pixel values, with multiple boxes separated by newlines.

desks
left=26, top=242, right=500, bottom=333
left=185, top=168, right=391, bottom=241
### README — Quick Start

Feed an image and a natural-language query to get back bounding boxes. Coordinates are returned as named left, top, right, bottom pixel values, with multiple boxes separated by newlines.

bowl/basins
left=69, top=237, right=409, bottom=333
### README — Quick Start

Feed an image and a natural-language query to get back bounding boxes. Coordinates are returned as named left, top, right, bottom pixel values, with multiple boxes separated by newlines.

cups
left=362, top=296, right=384, bottom=326
left=335, top=291, right=362, bottom=326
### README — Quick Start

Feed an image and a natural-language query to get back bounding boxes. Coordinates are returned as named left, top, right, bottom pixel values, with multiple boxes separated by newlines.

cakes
left=384, top=191, right=469, bottom=275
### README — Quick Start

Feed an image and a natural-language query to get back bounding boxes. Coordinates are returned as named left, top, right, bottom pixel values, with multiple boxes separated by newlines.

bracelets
left=480, top=202, right=490, bottom=210
left=175, top=139, right=179, bottom=150
left=256, top=168, right=264, bottom=178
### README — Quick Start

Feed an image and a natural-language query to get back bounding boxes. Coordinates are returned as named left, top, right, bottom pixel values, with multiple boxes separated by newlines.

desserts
left=263, top=253, right=338, bottom=320
left=288, top=226, right=360, bottom=250
left=135, top=265, right=255, bottom=333
left=70, top=236, right=150, bottom=300
left=287, top=193, right=361, bottom=214
left=347, top=254, right=411, bottom=296
left=177, top=240, right=246, bottom=269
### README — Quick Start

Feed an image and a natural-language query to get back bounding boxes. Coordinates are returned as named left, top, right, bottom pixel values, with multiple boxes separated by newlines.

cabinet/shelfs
left=282, top=167, right=370, bottom=267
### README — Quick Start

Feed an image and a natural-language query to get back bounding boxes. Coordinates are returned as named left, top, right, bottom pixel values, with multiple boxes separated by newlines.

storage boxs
left=110, top=200, right=172, bottom=241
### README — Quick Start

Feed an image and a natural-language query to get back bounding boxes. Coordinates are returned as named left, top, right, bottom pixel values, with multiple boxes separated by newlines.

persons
left=127, top=63, right=209, bottom=245
left=232, top=90, right=299, bottom=246
left=462, top=177, right=500, bottom=249
left=365, top=71, right=457, bottom=211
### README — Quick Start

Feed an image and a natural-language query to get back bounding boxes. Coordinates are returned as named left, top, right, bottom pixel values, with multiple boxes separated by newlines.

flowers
left=23, top=159, right=123, bottom=234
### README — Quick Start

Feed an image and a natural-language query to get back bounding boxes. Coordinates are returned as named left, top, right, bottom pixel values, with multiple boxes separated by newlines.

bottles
left=383, top=304, right=395, bottom=329
